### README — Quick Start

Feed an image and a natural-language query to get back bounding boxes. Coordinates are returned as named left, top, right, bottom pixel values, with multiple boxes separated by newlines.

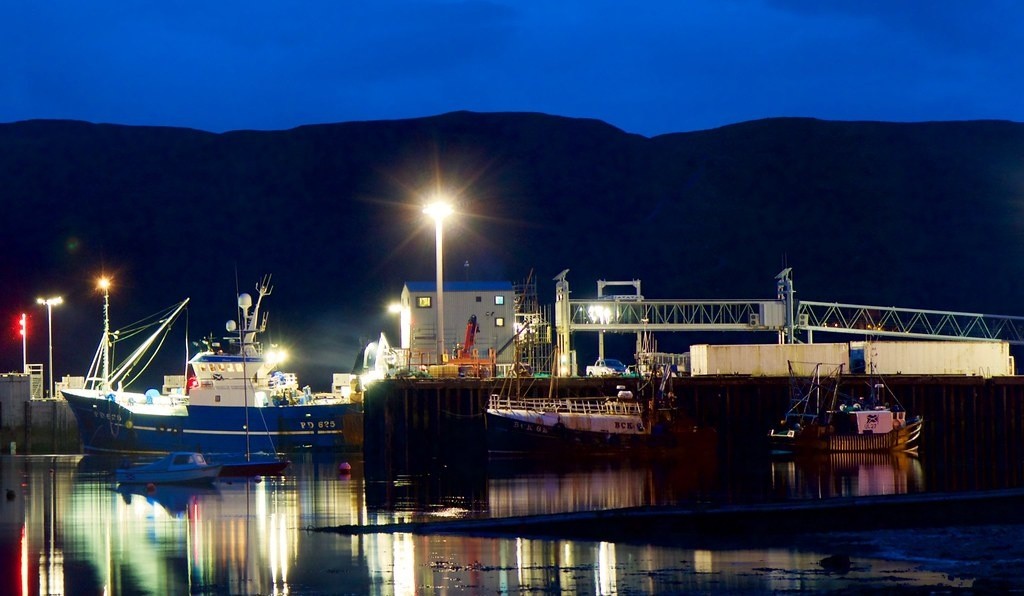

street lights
left=422, top=201, right=452, bottom=365
left=36, top=296, right=65, bottom=400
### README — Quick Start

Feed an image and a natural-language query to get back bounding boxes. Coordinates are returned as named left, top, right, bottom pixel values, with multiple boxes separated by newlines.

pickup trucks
left=586, top=359, right=626, bottom=377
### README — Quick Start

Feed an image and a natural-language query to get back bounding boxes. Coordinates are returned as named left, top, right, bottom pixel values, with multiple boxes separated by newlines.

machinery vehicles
left=444, top=314, right=494, bottom=378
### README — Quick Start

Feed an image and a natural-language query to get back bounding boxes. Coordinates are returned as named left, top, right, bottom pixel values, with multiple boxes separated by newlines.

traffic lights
left=19, top=314, right=32, bottom=336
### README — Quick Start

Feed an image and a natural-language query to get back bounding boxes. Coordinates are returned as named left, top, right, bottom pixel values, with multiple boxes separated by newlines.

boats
left=115, top=449, right=223, bottom=486
left=766, top=341, right=925, bottom=454
left=479, top=325, right=679, bottom=462
left=59, top=275, right=363, bottom=460
